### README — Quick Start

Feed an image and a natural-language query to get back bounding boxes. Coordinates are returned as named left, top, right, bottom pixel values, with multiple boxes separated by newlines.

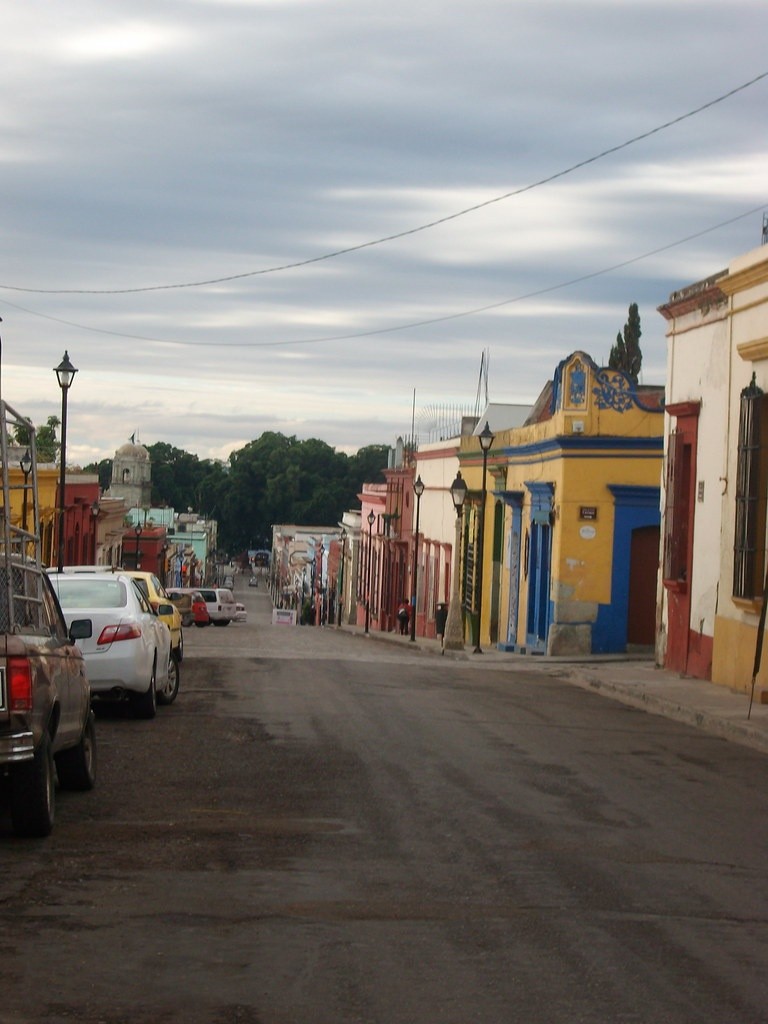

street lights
left=365, top=509, right=376, bottom=634
left=312, top=556, right=316, bottom=609
left=53, top=351, right=78, bottom=575
left=338, top=528, right=348, bottom=626
left=302, top=567, right=306, bottom=617
left=413, top=475, right=428, bottom=641
left=192, top=560, right=196, bottom=587
left=162, top=541, right=167, bottom=589
left=451, top=472, right=468, bottom=592
left=475, top=421, right=494, bottom=652
left=135, top=521, right=141, bottom=569
left=180, top=551, right=184, bottom=585
left=20, top=449, right=33, bottom=549
left=320, top=545, right=325, bottom=626
left=90, top=501, right=102, bottom=567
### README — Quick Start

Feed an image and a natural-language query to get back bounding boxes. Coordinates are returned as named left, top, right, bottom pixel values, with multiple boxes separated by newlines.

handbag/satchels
left=398, top=605, right=407, bottom=620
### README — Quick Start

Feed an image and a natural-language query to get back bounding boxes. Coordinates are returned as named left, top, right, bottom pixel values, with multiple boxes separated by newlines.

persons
left=399, top=599, right=411, bottom=634
left=437, top=605, right=447, bottom=640
left=280, top=600, right=283, bottom=608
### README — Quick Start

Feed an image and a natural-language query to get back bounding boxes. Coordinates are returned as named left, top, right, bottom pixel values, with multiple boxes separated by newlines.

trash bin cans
left=435, top=603, right=448, bottom=635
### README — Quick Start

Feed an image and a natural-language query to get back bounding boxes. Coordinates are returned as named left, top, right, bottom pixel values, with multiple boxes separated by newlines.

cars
left=234, top=603, right=248, bottom=623
left=106, top=570, right=184, bottom=663
left=167, top=590, right=210, bottom=627
left=48, top=574, right=179, bottom=718
left=223, top=576, right=258, bottom=590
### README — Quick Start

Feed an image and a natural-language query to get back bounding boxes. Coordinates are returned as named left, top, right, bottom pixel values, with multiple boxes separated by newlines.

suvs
left=0, top=556, right=99, bottom=830
left=190, top=588, right=238, bottom=628
